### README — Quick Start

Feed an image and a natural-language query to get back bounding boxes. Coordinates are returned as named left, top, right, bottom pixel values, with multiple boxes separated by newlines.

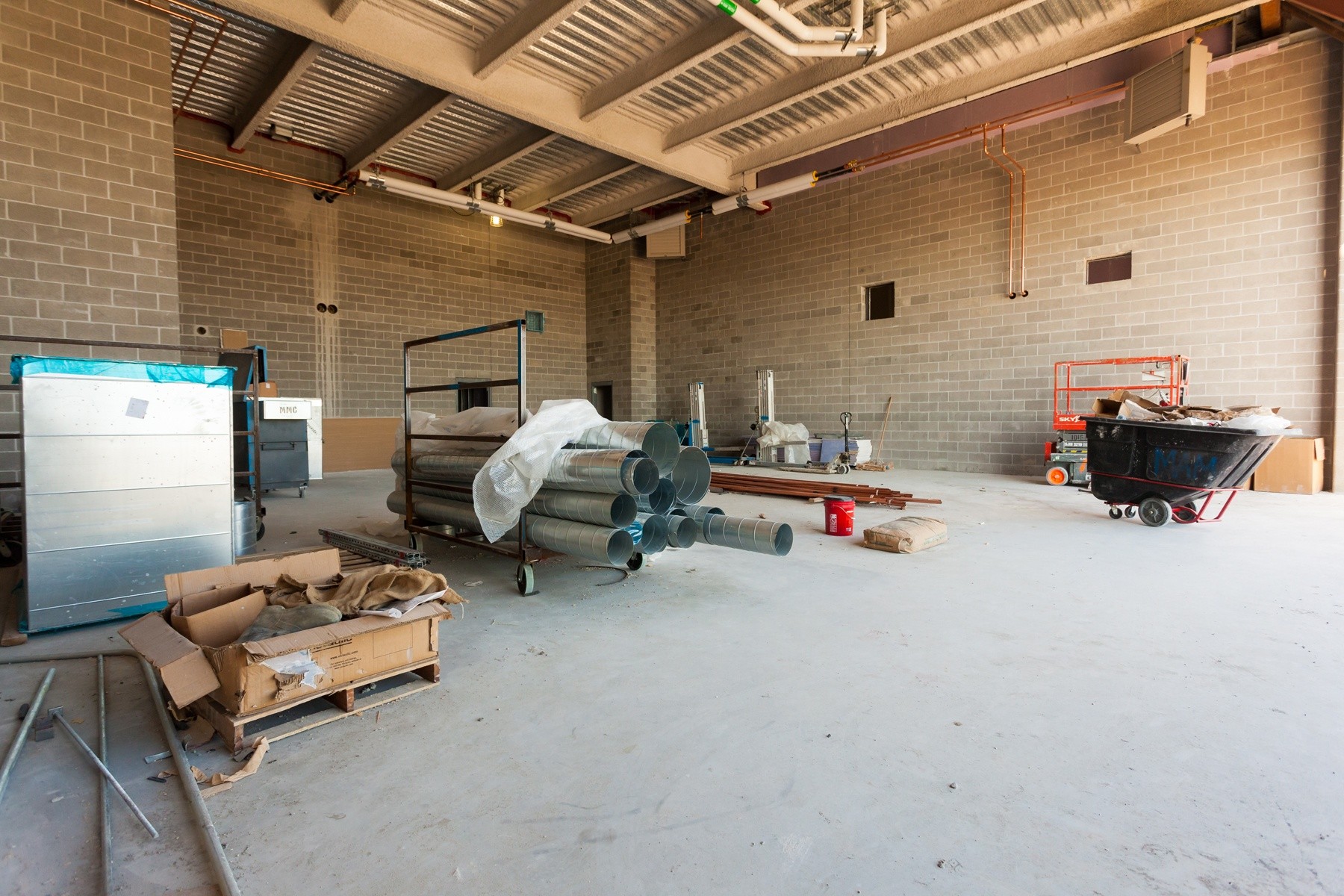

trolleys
left=732, top=411, right=854, bottom=473
left=1077, top=471, right=1246, bottom=528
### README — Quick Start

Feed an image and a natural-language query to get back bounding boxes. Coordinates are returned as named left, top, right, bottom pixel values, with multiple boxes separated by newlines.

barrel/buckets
left=823, top=494, right=855, bottom=536
left=235, top=502, right=258, bottom=558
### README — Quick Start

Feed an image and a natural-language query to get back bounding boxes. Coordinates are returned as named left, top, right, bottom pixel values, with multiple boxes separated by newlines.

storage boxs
left=0, top=566, right=18, bottom=644
left=117, top=547, right=450, bottom=710
left=1244, top=435, right=1325, bottom=495
left=248, top=382, right=278, bottom=397
left=1093, top=397, right=1123, bottom=420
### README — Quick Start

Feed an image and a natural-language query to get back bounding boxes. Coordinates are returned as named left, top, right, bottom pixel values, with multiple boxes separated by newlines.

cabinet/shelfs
left=245, top=397, right=322, bottom=490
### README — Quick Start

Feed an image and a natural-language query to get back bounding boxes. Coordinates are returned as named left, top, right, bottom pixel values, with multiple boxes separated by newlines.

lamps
left=490, top=214, right=503, bottom=227
